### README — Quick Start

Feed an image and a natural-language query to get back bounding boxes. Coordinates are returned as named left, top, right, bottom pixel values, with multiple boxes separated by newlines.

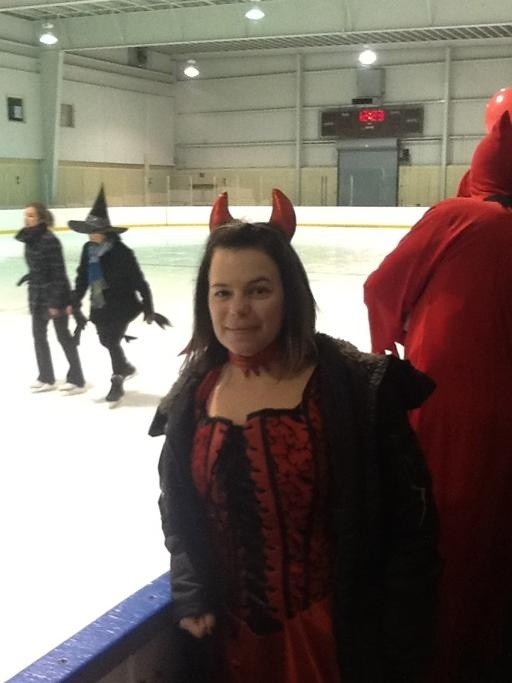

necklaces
left=224, top=343, right=281, bottom=378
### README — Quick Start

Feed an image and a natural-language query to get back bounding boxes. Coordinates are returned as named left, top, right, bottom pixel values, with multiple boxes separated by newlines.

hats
left=67, top=187, right=128, bottom=234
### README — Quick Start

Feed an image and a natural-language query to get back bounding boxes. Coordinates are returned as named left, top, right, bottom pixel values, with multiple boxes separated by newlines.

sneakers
left=30, top=379, right=45, bottom=387
left=60, top=384, right=76, bottom=389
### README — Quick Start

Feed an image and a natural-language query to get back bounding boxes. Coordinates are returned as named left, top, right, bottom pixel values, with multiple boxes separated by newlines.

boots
left=123, top=362, right=135, bottom=376
left=106, top=375, right=125, bottom=401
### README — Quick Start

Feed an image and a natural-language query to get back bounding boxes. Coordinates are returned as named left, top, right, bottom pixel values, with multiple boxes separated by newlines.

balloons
left=485, top=84, right=512, bottom=132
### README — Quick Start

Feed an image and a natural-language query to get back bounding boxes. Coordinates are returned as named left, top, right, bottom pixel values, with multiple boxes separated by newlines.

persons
left=143, top=186, right=458, bottom=681
left=367, top=106, right=512, bottom=681
left=64, top=225, right=172, bottom=402
left=13, top=200, right=82, bottom=394
